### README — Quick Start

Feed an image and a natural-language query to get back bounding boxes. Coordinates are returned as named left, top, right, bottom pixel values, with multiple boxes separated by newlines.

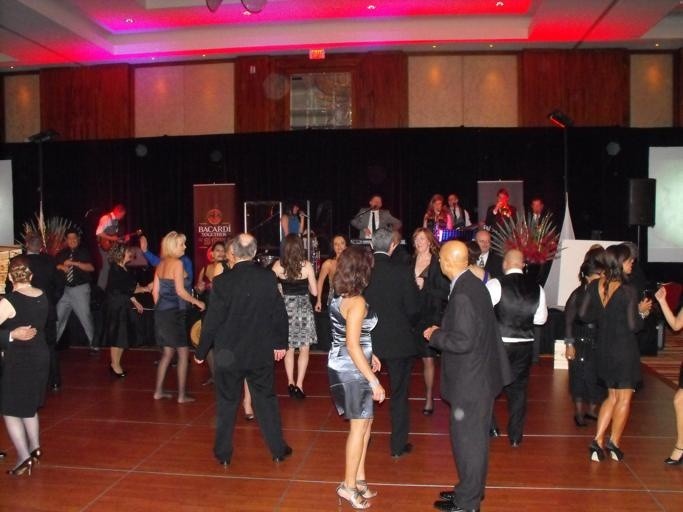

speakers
left=627, top=178, right=657, bottom=229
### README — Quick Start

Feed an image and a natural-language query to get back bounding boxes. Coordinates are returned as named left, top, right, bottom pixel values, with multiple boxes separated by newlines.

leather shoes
left=664, top=446, right=683, bottom=465
left=272, top=446, right=293, bottom=464
left=220, top=456, right=231, bottom=465
left=490, top=428, right=501, bottom=439
left=509, top=437, right=521, bottom=449
left=245, top=413, right=255, bottom=422
left=390, top=443, right=414, bottom=460
left=573, top=414, right=587, bottom=427
left=440, top=489, right=485, bottom=503
left=434, top=499, right=480, bottom=512
left=585, top=412, right=600, bottom=421
left=51, top=381, right=61, bottom=389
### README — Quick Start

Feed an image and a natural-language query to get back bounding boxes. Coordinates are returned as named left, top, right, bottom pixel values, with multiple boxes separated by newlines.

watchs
left=428, top=333, right=432, bottom=337
left=77, top=261, right=80, bottom=267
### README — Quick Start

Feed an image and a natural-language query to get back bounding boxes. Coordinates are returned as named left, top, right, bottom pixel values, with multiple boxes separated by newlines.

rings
left=568, top=356, right=571, bottom=359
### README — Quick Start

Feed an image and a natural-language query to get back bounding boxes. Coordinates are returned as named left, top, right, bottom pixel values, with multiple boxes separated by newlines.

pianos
left=350, top=239, right=406, bottom=245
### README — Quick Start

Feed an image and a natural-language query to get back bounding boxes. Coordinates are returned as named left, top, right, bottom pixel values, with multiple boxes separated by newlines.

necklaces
left=11, top=284, right=33, bottom=292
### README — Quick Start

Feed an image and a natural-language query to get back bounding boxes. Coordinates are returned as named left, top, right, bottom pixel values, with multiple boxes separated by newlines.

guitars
left=100, top=228, right=142, bottom=252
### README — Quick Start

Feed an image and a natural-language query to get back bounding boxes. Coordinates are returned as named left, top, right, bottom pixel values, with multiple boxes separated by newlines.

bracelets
left=143, top=286, right=146, bottom=293
left=369, top=379, right=380, bottom=388
left=566, top=343, right=573, bottom=347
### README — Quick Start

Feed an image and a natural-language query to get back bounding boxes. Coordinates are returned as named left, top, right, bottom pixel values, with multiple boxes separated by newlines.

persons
left=409, top=228, right=452, bottom=417
left=0, top=325, right=38, bottom=460
left=475, top=229, right=504, bottom=278
left=197, top=240, right=229, bottom=385
left=281, top=203, right=304, bottom=239
left=526, top=199, right=554, bottom=230
left=486, top=249, right=548, bottom=448
left=424, top=194, right=453, bottom=233
left=564, top=257, right=605, bottom=428
left=655, top=286, right=683, bottom=466
left=152, top=230, right=206, bottom=403
left=351, top=195, right=402, bottom=239
left=139, top=236, right=194, bottom=368
left=270, top=233, right=317, bottom=399
left=422, top=240, right=515, bottom=511
left=314, top=233, right=349, bottom=313
left=585, top=244, right=606, bottom=263
left=329, top=245, right=385, bottom=510
left=448, top=193, right=471, bottom=230
left=214, top=239, right=255, bottom=423
left=55, top=228, right=100, bottom=357
left=99, top=240, right=150, bottom=378
left=96, top=204, right=130, bottom=305
left=362, top=228, right=424, bottom=459
left=193, top=234, right=295, bottom=466
left=578, top=244, right=653, bottom=462
left=0, top=256, right=51, bottom=476
left=466, top=240, right=491, bottom=285
left=6, top=233, right=65, bottom=392
left=487, top=188, right=517, bottom=236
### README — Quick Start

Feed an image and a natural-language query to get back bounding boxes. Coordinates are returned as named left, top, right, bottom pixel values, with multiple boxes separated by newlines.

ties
left=66, top=252, right=74, bottom=285
left=479, top=258, right=483, bottom=268
left=371, top=212, right=376, bottom=234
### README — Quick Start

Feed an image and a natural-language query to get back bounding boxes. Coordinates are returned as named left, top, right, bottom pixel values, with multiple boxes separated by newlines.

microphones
left=302, top=213, right=311, bottom=219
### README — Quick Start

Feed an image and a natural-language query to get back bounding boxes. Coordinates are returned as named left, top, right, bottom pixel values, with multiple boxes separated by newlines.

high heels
left=7, top=456, right=34, bottom=477
left=109, top=368, right=127, bottom=379
left=604, top=441, right=624, bottom=462
left=32, top=447, right=44, bottom=467
left=354, top=480, right=378, bottom=499
left=335, top=482, right=372, bottom=511
left=588, top=441, right=605, bottom=464
left=422, top=397, right=435, bottom=416
left=287, top=383, right=306, bottom=400
